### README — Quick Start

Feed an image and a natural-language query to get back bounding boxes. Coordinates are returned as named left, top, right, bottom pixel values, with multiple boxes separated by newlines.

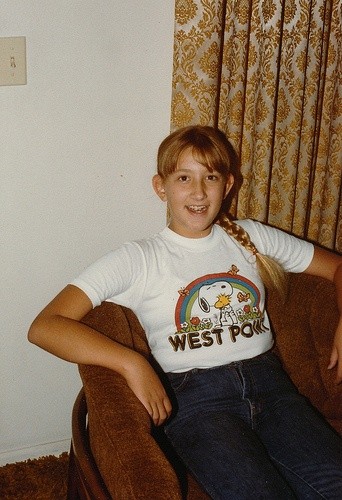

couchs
left=68, top=269, right=342, bottom=500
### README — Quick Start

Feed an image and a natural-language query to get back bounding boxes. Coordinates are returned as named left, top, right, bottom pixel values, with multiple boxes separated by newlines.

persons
left=27, top=125, right=342, bottom=500
